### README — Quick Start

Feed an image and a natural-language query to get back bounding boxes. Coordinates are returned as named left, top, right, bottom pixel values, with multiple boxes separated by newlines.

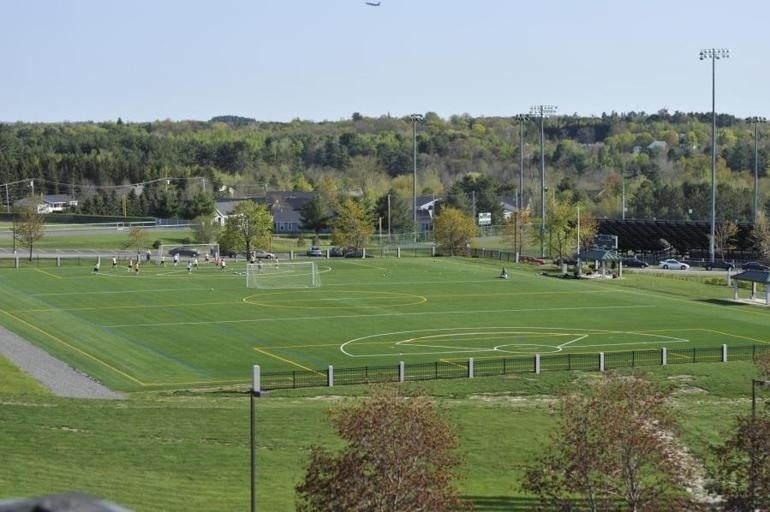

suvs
left=209, top=245, right=236, bottom=257
left=518, top=255, right=544, bottom=265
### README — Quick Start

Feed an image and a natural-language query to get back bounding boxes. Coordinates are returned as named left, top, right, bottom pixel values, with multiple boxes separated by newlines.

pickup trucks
left=702, top=259, right=735, bottom=272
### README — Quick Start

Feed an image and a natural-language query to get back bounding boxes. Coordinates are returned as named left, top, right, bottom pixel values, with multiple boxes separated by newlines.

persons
left=92, top=252, right=281, bottom=274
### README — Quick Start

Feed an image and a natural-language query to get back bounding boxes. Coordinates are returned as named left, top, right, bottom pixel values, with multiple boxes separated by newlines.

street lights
left=700, top=48, right=729, bottom=259
left=512, top=113, right=535, bottom=256
left=526, top=104, right=560, bottom=261
left=248, top=388, right=272, bottom=511
left=742, top=115, right=769, bottom=230
left=404, top=113, right=427, bottom=237
left=751, top=377, right=770, bottom=512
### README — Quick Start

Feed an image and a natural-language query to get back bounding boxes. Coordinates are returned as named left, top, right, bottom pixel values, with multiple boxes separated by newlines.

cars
left=551, top=255, right=580, bottom=267
left=328, top=247, right=344, bottom=258
left=306, top=246, right=321, bottom=255
left=252, top=249, right=275, bottom=258
left=742, top=262, right=768, bottom=272
left=168, top=247, right=199, bottom=257
left=659, top=258, right=690, bottom=270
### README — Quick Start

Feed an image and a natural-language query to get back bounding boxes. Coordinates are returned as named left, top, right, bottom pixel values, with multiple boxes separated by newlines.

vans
left=622, top=258, right=650, bottom=268
